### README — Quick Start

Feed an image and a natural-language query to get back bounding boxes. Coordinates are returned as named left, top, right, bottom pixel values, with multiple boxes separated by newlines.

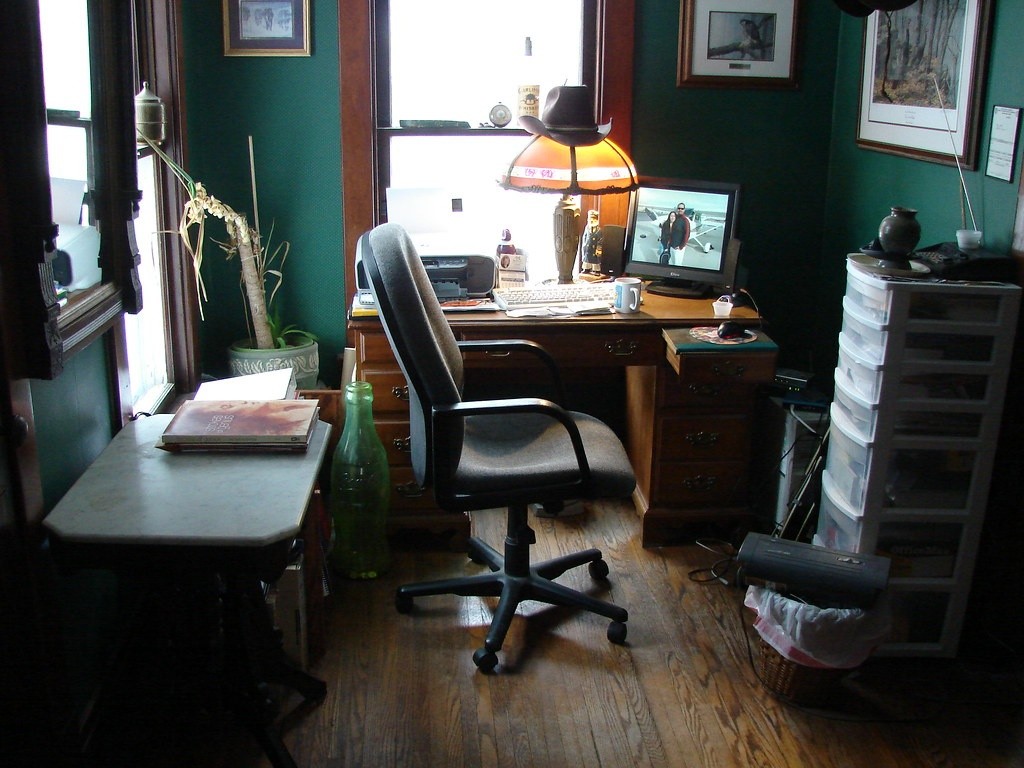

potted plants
left=136, top=125, right=319, bottom=390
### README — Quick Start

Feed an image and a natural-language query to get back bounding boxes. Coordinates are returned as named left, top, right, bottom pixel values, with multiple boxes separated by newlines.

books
left=348, top=298, right=378, bottom=320
left=162, top=400, right=321, bottom=453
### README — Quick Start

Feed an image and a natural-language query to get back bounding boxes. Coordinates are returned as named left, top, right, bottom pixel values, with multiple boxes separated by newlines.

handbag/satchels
left=731, top=530, right=891, bottom=608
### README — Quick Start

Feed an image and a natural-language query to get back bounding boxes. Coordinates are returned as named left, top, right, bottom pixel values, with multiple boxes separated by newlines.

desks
left=44, top=412, right=331, bottom=768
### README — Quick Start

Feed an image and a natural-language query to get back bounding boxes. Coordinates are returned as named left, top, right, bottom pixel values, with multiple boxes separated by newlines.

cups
left=613, top=277, right=642, bottom=314
left=957, top=230, right=982, bottom=249
left=712, top=301, right=733, bottom=316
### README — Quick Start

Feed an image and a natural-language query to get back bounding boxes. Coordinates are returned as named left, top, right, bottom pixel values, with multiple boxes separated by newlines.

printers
left=354, top=199, right=496, bottom=305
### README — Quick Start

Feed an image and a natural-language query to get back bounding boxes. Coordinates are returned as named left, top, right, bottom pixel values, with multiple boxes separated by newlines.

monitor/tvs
left=621, top=175, right=742, bottom=298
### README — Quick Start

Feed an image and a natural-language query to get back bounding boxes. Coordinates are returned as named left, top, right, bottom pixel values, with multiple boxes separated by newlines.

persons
left=580, top=210, right=603, bottom=276
left=502, top=256, right=510, bottom=268
left=662, top=203, right=689, bottom=265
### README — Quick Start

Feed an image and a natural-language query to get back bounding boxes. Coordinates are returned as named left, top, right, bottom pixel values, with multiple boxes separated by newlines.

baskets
left=751, top=628, right=878, bottom=715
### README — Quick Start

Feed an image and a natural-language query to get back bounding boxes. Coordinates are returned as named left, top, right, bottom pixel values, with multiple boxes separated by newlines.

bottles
left=516, top=37, right=540, bottom=126
left=878, top=208, right=921, bottom=270
left=134, top=82, right=165, bottom=146
left=495, top=229, right=517, bottom=288
left=325, top=382, right=392, bottom=581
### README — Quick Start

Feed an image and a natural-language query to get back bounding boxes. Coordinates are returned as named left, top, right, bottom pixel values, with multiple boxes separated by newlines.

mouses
left=717, top=321, right=744, bottom=339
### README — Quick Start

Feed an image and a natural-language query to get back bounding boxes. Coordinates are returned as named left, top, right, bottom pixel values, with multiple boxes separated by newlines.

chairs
left=353, top=222, right=635, bottom=671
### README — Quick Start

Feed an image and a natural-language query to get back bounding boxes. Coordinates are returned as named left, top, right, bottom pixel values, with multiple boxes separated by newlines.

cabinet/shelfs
left=800, top=252, right=1023, bottom=695
left=346, top=323, right=779, bottom=550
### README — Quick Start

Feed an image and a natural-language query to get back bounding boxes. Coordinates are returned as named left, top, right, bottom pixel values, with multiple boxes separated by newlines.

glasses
left=678, top=207, right=684, bottom=210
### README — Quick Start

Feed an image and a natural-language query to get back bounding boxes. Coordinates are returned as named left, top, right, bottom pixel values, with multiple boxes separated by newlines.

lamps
left=500, top=117, right=640, bottom=284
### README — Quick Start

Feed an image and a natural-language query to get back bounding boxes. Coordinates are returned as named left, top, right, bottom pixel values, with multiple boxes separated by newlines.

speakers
left=716, top=239, right=745, bottom=307
left=601, top=225, right=626, bottom=277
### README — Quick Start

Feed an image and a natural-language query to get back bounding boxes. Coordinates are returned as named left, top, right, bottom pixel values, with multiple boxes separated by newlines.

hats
left=523, top=86, right=613, bottom=147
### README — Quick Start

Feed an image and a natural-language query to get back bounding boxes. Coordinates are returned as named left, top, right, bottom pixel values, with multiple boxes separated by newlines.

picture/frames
left=856, top=1, right=992, bottom=169
left=222, top=1, right=311, bottom=57
left=678, top=1, right=805, bottom=86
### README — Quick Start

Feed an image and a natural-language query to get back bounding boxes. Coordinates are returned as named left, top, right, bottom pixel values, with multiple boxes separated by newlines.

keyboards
left=491, top=282, right=643, bottom=310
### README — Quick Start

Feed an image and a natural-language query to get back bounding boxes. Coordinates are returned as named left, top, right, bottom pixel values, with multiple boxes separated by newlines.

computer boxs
left=775, top=407, right=830, bottom=538
